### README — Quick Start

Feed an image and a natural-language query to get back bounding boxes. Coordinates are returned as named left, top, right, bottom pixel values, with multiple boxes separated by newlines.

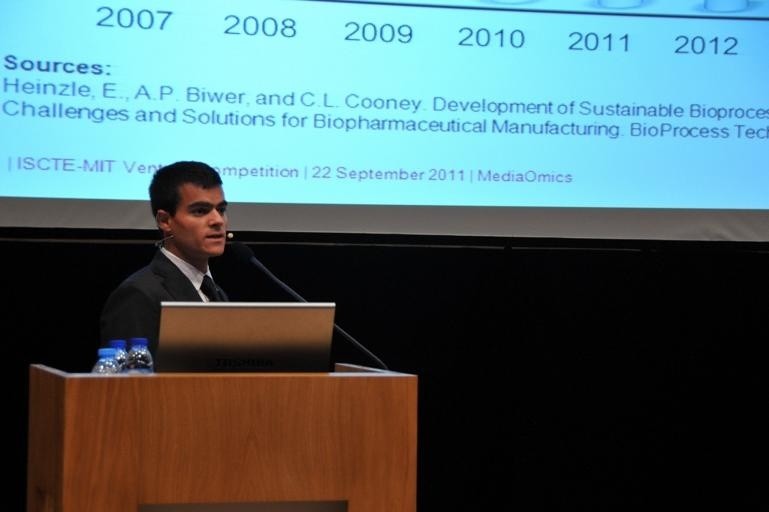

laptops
left=156, top=301, right=336, bottom=371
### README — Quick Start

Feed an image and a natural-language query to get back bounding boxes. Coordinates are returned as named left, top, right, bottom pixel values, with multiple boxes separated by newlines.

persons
left=94, top=160, right=231, bottom=373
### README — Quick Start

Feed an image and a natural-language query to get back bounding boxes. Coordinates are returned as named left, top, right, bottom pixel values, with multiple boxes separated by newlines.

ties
left=199, top=275, right=222, bottom=302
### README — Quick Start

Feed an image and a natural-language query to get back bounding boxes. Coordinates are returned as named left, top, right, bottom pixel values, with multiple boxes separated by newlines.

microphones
left=230, top=241, right=388, bottom=370
left=228, top=232, right=234, bottom=239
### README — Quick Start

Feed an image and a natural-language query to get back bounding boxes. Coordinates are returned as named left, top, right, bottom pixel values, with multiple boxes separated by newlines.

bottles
left=91, top=337, right=155, bottom=374
